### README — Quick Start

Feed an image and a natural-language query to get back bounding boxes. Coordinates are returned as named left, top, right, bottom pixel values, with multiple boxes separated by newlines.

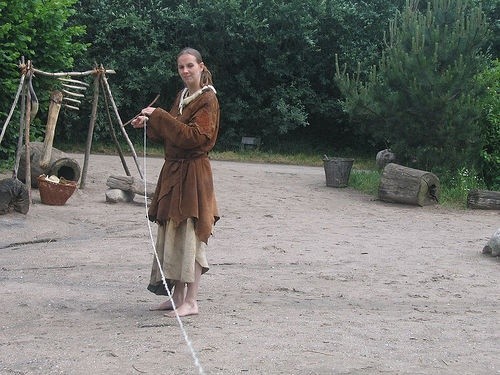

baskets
left=322, top=158, right=355, bottom=187
left=37, top=176, right=76, bottom=205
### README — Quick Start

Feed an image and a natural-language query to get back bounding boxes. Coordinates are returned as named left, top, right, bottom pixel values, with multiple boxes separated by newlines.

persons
left=130, top=48, right=221, bottom=315
